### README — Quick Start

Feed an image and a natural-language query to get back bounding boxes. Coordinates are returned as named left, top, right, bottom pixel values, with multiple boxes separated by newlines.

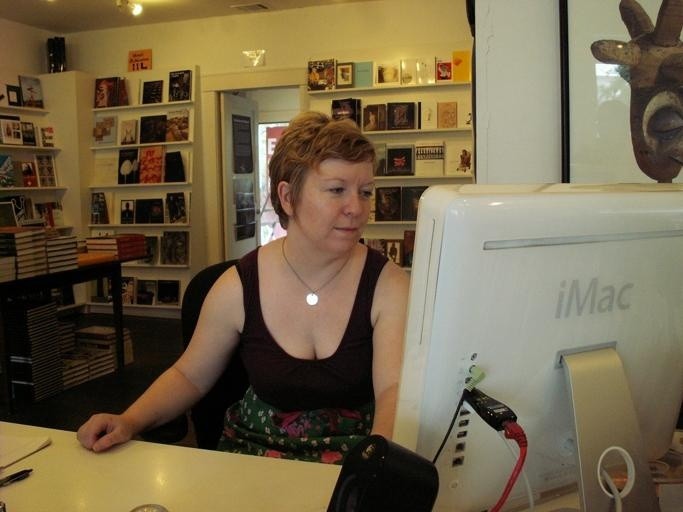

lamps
left=114, top=0, right=149, bottom=19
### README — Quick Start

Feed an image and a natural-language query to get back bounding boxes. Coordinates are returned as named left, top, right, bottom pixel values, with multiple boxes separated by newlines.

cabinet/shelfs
left=0, top=101, right=71, bottom=237
left=305, top=77, right=474, bottom=272
left=81, top=66, right=198, bottom=311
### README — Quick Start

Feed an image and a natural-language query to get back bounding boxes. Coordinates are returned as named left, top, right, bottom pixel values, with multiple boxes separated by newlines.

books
left=0, top=222, right=146, bottom=392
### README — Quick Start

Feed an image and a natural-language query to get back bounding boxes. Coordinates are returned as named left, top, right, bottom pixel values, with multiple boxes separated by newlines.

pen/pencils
left=0, top=468, right=33, bottom=488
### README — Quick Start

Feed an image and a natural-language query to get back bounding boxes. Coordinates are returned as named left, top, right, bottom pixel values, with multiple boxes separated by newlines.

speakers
left=327, top=434, right=439, bottom=512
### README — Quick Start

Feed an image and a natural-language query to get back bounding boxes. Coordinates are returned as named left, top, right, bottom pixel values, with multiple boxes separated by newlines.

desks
left=1, top=424, right=682, bottom=512
left=0, top=249, right=153, bottom=373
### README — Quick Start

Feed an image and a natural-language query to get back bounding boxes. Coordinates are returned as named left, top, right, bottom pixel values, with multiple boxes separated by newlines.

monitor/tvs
left=392, top=183, right=683, bottom=512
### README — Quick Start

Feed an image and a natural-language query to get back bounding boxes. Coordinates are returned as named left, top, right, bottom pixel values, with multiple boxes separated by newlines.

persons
left=77, top=110, right=410, bottom=466
left=123, top=203, right=133, bottom=221
left=365, top=111, right=376, bottom=131
left=386, top=243, right=398, bottom=262
left=310, top=68, right=318, bottom=89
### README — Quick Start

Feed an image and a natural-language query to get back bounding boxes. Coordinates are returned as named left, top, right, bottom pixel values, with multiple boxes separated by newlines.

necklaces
left=278, top=237, right=351, bottom=306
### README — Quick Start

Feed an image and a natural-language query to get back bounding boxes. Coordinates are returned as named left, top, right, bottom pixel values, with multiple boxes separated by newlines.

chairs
left=178, top=257, right=252, bottom=449
